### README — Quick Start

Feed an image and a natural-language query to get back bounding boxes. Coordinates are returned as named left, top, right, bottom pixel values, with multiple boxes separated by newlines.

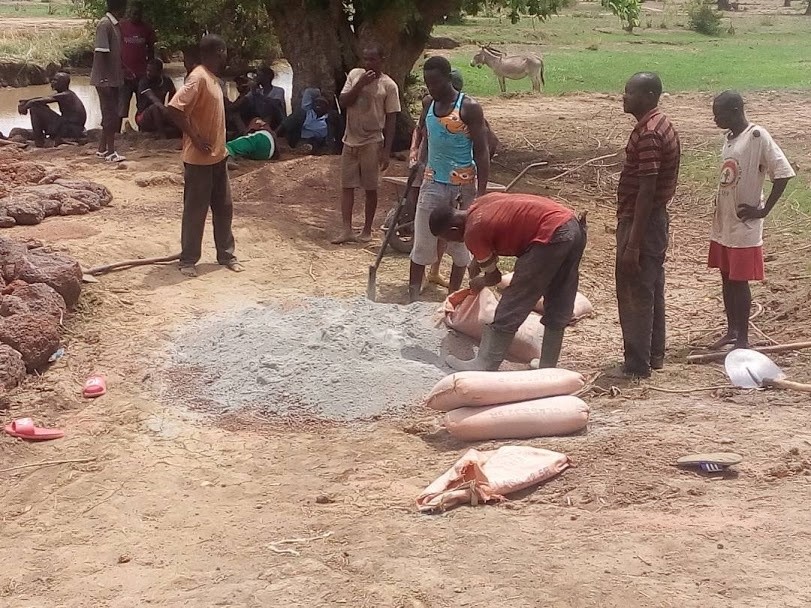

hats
left=451, top=67, right=464, bottom=90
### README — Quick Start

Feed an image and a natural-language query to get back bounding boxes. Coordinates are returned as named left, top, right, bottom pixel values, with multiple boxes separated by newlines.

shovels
left=725, top=348, right=811, bottom=393
left=368, top=159, right=421, bottom=301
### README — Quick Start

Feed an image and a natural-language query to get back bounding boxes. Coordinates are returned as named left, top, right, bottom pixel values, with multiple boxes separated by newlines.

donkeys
left=470, top=41, right=546, bottom=94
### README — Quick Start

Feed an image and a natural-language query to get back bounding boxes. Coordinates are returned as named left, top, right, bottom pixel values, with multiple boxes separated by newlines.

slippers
left=676, top=452, right=742, bottom=474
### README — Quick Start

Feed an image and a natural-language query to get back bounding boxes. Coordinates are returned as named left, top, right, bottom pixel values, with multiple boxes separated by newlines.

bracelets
left=100, top=79, right=108, bottom=82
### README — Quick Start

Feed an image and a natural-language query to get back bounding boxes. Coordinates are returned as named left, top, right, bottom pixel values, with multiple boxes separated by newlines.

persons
left=89, top=0, right=330, bottom=170
left=612, top=72, right=681, bottom=377
left=167, top=33, right=245, bottom=276
left=331, top=41, right=401, bottom=243
left=430, top=193, right=586, bottom=372
left=409, top=56, right=499, bottom=305
left=18, top=73, right=86, bottom=148
left=708, top=90, right=797, bottom=350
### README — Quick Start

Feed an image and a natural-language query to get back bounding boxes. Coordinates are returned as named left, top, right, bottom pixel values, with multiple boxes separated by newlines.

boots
left=530, top=326, right=563, bottom=369
left=445, top=324, right=515, bottom=373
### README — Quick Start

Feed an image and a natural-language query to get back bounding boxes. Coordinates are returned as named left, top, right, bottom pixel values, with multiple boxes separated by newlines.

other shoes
left=300, top=143, right=313, bottom=154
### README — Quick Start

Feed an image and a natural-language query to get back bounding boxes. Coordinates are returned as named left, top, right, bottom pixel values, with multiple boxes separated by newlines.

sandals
left=105, top=151, right=126, bottom=162
left=96, top=151, right=108, bottom=158
